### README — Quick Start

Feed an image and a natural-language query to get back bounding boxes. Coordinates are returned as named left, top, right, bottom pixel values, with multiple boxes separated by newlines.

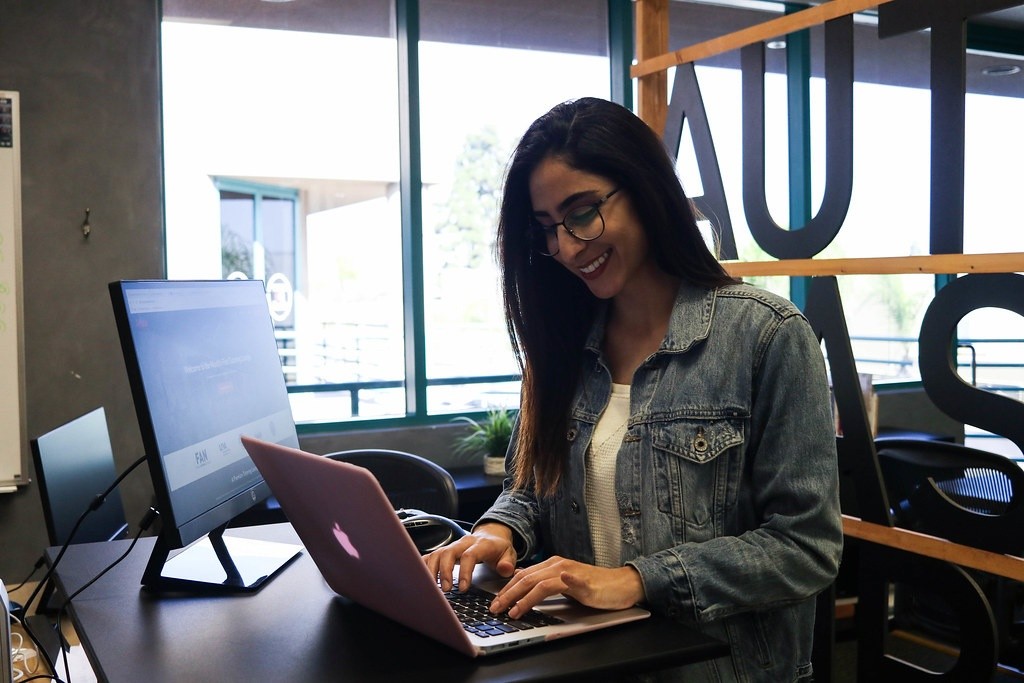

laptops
left=241, top=435, right=652, bottom=656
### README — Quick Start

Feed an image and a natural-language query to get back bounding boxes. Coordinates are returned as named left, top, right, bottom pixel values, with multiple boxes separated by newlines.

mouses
left=399, top=515, right=466, bottom=557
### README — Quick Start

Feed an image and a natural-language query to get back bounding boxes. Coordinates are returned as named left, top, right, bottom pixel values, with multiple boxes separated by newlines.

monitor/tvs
left=109, top=277, right=302, bottom=593
left=34, top=407, right=130, bottom=615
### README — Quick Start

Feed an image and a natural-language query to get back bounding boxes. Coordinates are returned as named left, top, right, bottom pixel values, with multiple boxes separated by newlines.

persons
left=420, top=97, right=844, bottom=683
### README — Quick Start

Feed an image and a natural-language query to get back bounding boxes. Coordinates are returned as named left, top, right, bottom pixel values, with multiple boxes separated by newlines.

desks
left=40, top=515, right=731, bottom=683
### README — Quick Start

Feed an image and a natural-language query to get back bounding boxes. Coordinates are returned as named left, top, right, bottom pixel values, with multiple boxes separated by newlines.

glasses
left=524, top=185, right=624, bottom=256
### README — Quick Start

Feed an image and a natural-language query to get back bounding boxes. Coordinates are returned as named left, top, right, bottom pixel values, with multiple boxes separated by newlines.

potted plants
left=447, top=405, right=517, bottom=475
